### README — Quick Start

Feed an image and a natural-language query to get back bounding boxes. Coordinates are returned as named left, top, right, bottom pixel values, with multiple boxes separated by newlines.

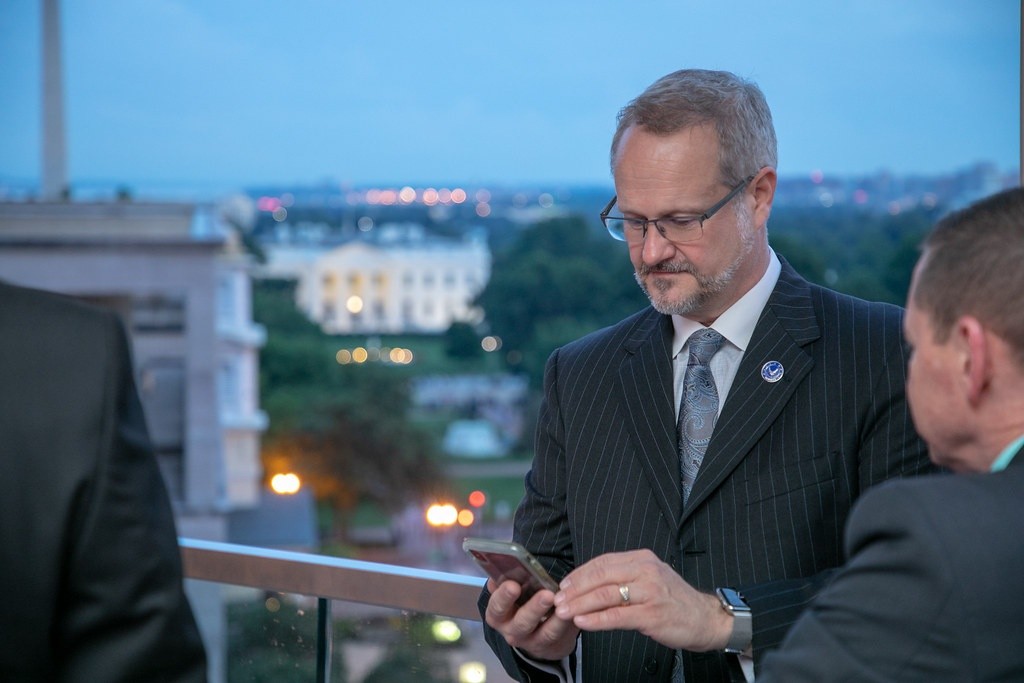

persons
left=474, top=70, right=941, bottom=683
left=755, top=188, right=1024, bottom=683
left=0, top=279, right=211, bottom=683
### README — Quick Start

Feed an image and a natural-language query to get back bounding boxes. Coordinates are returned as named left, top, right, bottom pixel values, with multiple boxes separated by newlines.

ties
left=672, top=329, right=727, bottom=683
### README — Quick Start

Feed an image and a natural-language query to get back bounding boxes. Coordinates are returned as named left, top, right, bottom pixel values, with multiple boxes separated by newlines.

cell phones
left=462, top=538, right=560, bottom=616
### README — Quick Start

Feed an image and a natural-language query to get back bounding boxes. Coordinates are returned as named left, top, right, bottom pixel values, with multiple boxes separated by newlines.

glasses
left=600, top=174, right=757, bottom=245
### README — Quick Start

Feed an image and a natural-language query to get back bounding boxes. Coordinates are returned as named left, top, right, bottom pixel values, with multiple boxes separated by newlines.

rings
left=619, top=584, right=630, bottom=607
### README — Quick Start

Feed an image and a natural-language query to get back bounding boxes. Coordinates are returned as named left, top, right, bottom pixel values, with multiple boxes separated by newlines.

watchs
left=714, top=587, right=752, bottom=656
